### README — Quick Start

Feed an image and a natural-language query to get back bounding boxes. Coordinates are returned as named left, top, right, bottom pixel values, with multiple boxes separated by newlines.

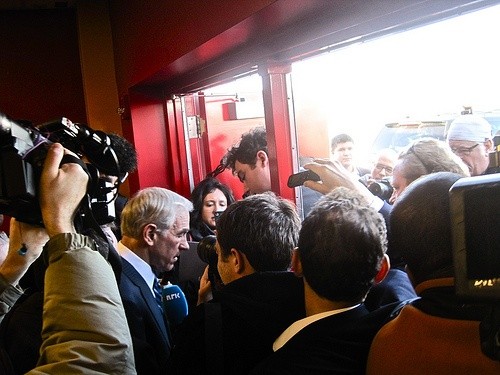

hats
left=446, top=115, right=492, bottom=141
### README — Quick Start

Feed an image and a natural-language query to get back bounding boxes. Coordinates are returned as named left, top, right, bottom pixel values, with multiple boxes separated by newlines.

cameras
left=196, top=235, right=222, bottom=287
left=366, top=176, right=394, bottom=200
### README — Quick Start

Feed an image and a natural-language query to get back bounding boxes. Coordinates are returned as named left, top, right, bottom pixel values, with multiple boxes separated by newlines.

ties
left=152, top=278, right=167, bottom=323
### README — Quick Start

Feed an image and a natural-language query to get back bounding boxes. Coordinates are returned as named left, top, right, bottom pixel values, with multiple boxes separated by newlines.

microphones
left=54, top=123, right=137, bottom=176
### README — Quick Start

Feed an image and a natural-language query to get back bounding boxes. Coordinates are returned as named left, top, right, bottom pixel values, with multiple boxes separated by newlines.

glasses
left=375, top=163, right=394, bottom=174
left=451, top=142, right=480, bottom=156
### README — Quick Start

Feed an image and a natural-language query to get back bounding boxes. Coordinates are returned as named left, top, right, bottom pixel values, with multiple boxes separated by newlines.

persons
left=245, top=187, right=391, bottom=375
left=162, top=112, right=500, bottom=312
left=117, top=187, right=194, bottom=375
left=365, top=171, right=500, bottom=375
left=0, top=111, right=137, bottom=375
left=161, top=194, right=304, bottom=375
